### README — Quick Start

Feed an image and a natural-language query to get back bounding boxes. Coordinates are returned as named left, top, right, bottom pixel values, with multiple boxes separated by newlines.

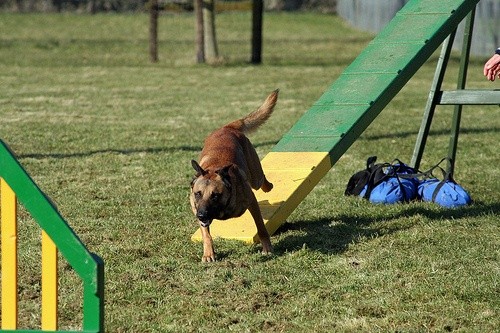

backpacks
left=345, top=157, right=417, bottom=203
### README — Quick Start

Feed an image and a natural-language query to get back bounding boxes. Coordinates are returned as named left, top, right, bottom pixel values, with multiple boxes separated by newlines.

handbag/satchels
left=419, top=157, right=472, bottom=209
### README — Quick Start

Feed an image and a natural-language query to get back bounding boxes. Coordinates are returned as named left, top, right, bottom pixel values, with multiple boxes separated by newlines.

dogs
left=188, top=89, right=279, bottom=264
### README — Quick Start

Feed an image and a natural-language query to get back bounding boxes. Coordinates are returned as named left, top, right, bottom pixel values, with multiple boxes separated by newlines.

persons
left=483, top=47, right=500, bottom=81
